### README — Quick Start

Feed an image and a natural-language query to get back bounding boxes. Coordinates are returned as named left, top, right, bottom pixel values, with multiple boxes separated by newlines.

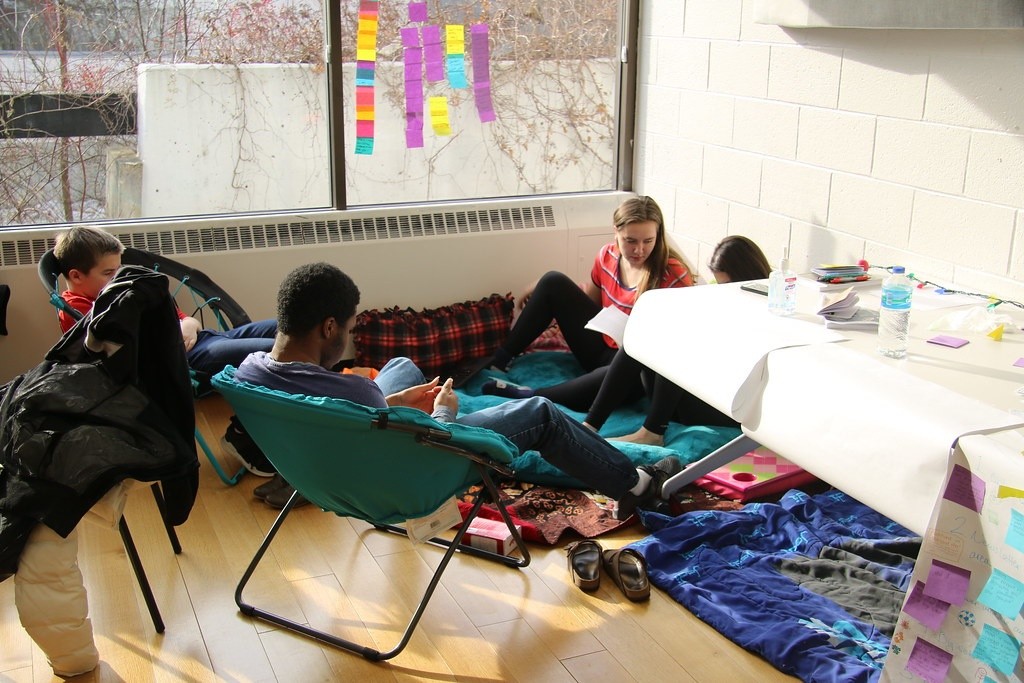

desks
left=625, top=274, right=1024, bottom=539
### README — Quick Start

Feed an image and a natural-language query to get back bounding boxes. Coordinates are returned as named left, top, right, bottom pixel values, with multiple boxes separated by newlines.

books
left=819, top=288, right=880, bottom=330
left=813, top=264, right=865, bottom=277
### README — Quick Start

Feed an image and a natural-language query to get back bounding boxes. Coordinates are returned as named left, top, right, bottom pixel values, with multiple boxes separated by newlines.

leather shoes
left=618, top=456, right=682, bottom=513
left=264, top=485, right=310, bottom=509
left=253, top=474, right=289, bottom=500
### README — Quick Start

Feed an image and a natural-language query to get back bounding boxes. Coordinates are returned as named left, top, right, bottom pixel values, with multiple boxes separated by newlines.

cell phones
left=740, top=282, right=769, bottom=296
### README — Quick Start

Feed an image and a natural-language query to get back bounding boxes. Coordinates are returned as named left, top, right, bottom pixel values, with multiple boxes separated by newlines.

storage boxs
left=461, top=516, right=522, bottom=557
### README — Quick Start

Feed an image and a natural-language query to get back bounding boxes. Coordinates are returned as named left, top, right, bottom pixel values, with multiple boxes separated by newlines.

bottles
left=877, top=265, right=913, bottom=358
left=767, top=258, right=799, bottom=316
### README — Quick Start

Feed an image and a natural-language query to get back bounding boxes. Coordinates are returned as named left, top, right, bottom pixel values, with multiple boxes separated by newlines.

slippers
left=602, top=547, right=649, bottom=600
left=568, top=539, right=603, bottom=591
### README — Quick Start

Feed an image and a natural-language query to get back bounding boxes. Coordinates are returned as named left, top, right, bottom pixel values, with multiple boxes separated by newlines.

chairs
left=37, top=246, right=251, bottom=485
left=210, top=365, right=531, bottom=661
left=64, top=271, right=186, bottom=634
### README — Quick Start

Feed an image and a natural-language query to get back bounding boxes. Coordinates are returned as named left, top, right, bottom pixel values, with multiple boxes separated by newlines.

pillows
left=349, top=290, right=516, bottom=378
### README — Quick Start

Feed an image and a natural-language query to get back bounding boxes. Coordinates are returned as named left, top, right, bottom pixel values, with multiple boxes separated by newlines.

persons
left=53, top=228, right=278, bottom=476
left=579, top=235, right=772, bottom=446
left=233, top=262, right=683, bottom=508
left=483, top=196, right=693, bottom=414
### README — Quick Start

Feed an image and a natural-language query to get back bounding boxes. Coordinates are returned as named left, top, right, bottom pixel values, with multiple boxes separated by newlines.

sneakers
left=220, top=424, right=277, bottom=477
